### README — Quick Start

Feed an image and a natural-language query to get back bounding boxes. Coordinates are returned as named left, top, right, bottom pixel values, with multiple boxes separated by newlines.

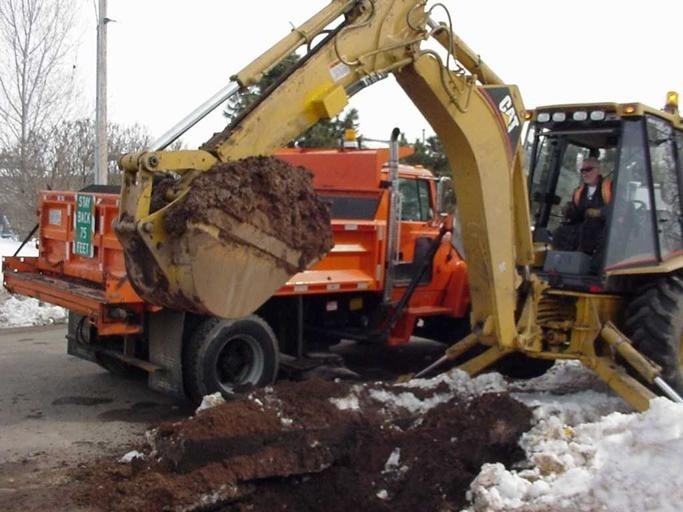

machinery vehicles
left=109, top=0, right=683, bottom=416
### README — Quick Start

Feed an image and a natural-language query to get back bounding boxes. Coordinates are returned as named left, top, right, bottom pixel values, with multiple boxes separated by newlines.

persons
left=552, top=157, right=624, bottom=255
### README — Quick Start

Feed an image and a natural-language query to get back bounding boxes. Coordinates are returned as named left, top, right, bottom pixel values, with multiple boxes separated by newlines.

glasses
left=580, top=167, right=596, bottom=173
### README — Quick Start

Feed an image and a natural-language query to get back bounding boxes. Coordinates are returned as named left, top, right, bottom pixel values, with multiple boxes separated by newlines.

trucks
left=6, top=131, right=478, bottom=407
